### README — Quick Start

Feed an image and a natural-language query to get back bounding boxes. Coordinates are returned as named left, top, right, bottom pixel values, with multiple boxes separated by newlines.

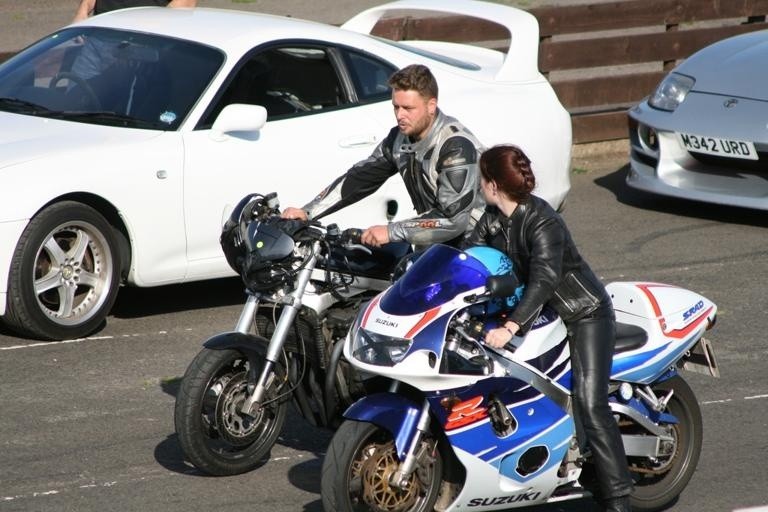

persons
left=56, top=2, right=200, bottom=120
left=464, top=144, right=636, bottom=512
left=280, top=63, right=489, bottom=255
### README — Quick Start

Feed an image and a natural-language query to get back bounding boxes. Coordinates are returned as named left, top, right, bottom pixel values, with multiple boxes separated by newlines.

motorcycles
left=173, top=193, right=412, bottom=477
left=322, top=245, right=716, bottom=512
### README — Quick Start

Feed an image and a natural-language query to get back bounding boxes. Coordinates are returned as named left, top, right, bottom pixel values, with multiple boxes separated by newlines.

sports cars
left=626, top=27, right=768, bottom=212
left=0, top=0, right=572, bottom=342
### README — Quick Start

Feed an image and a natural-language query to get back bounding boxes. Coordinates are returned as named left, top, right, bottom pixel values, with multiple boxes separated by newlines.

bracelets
left=500, top=324, right=514, bottom=339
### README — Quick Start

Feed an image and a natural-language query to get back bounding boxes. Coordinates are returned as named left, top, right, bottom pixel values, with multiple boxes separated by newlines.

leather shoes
left=602, top=495, right=631, bottom=512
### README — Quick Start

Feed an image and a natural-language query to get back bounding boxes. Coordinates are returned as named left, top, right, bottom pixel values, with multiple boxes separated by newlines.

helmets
left=450, top=246, right=526, bottom=317
left=219, top=193, right=266, bottom=276
